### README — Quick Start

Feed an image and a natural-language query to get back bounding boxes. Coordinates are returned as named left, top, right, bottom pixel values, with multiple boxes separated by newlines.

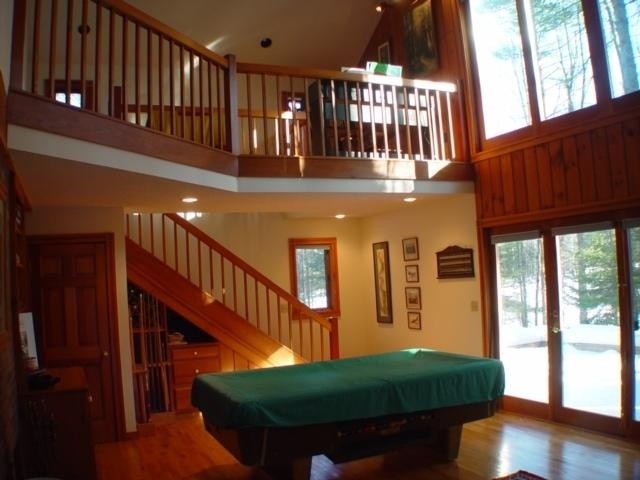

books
left=343, top=61, right=404, bottom=78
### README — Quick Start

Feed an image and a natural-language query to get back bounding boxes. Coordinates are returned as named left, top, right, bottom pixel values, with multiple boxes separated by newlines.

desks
left=191, top=348, right=503, bottom=480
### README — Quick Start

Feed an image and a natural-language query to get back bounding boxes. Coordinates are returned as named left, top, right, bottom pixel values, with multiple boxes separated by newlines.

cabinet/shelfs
left=16, top=390, right=97, bottom=480
left=167, top=344, right=221, bottom=413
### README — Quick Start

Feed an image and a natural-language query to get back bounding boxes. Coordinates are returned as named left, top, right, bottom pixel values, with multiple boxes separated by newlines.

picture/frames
left=372, top=241, right=394, bottom=324
left=401, top=1, right=442, bottom=79
left=401, top=236, right=423, bottom=331
left=376, top=36, right=393, bottom=64
